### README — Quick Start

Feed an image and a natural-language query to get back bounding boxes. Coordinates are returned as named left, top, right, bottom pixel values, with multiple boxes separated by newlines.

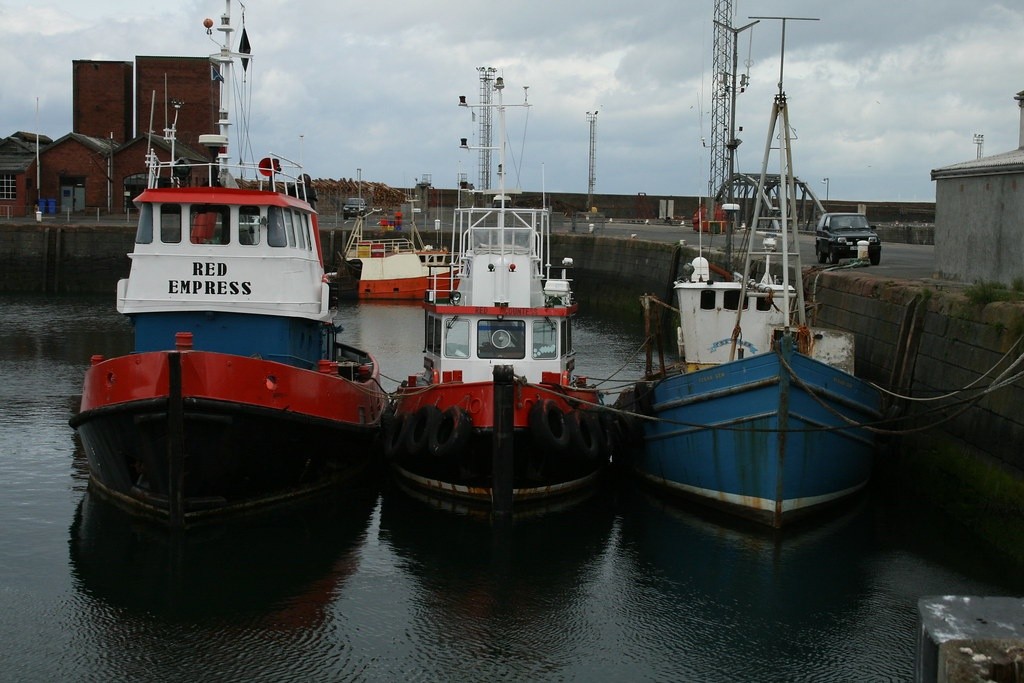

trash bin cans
left=38, top=198, right=57, bottom=215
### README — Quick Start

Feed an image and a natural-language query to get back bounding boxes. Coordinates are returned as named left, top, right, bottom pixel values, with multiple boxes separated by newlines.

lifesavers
left=384, top=414, right=409, bottom=457
left=527, top=399, right=571, bottom=448
left=567, top=411, right=599, bottom=458
left=428, top=405, right=474, bottom=459
left=406, top=405, right=441, bottom=455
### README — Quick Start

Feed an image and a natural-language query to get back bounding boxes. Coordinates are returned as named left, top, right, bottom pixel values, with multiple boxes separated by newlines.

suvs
left=814, top=213, right=885, bottom=267
left=342, top=196, right=369, bottom=221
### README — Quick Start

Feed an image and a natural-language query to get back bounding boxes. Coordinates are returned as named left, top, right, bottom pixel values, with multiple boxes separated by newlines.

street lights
left=821, top=177, right=829, bottom=212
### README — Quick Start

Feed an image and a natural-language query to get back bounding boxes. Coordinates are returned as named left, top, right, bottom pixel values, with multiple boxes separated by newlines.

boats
left=66, top=0, right=913, bottom=549
